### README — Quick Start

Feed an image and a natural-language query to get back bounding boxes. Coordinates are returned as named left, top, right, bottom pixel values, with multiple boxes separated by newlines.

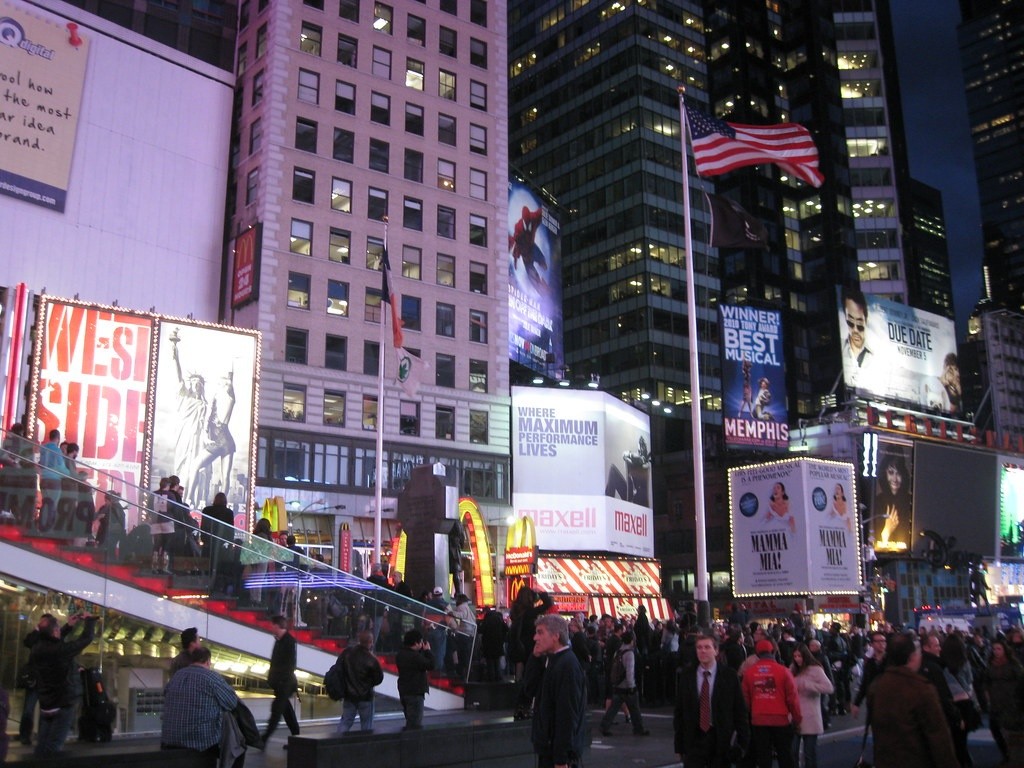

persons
left=738, top=349, right=776, bottom=421
left=874, top=442, right=911, bottom=552
left=757, top=481, right=795, bottom=535
left=604, top=436, right=649, bottom=507
left=922, top=353, right=963, bottom=415
left=839, top=286, right=881, bottom=393
left=969, top=563, right=991, bottom=615
left=170, top=347, right=235, bottom=510
left=826, top=483, right=852, bottom=533
left=0, top=422, right=1024, bottom=768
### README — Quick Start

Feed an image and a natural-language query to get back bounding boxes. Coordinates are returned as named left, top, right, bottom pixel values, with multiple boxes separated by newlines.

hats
left=756, top=639, right=773, bottom=653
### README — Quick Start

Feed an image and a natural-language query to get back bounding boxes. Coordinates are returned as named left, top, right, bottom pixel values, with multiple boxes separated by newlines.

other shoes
left=633, top=730, right=650, bottom=736
left=598, top=727, right=613, bottom=737
left=829, top=708, right=839, bottom=716
left=823, top=722, right=831, bottom=727
left=839, top=705, right=847, bottom=715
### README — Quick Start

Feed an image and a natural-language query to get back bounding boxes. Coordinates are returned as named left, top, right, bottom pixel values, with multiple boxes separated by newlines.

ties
left=700, top=671, right=712, bottom=733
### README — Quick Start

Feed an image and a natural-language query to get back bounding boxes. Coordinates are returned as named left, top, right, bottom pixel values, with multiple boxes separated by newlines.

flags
left=382, top=245, right=403, bottom=348
left=681, top=98, right=824, bottom=188
left=395, top=347, right=428, bottom=397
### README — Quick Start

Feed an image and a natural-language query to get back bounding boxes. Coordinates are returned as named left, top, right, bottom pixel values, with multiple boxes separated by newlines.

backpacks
left=610, top=648, right=633, bottom=684
left=324, top=647, right=351, bottom=700
